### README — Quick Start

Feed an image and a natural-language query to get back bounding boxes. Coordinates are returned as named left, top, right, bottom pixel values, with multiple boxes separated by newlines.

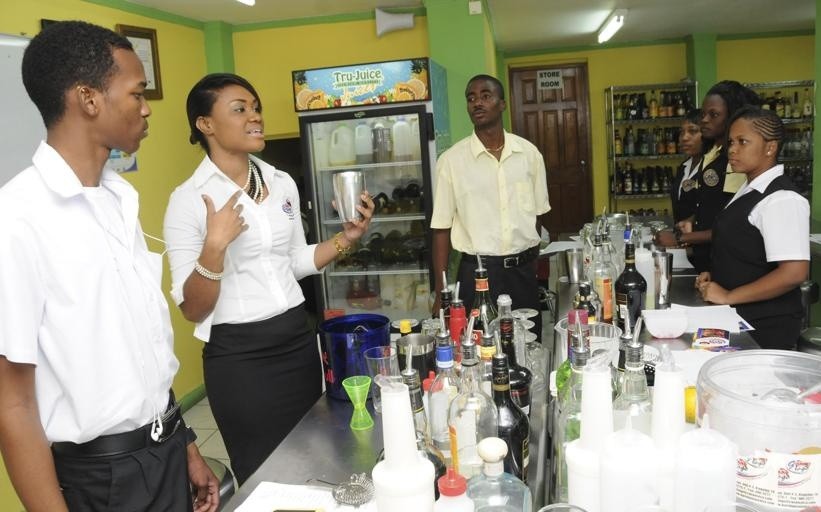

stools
left=800, top=327, right=821, bottom=355
left=800, top=281, right=818, bottom=329
left=190, top=456, right=234, bottom=512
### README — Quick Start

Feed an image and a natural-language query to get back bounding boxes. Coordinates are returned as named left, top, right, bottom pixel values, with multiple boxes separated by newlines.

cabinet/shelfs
left=604, top=81, right=699, bottom=213
left=744, top=80, right=815, bottom=199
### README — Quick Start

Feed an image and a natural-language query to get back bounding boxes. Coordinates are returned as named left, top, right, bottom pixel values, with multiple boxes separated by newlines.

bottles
left=759, top=89, right=813, bottom=188
left=611, top=91, right=692, bottom=195
left=334, top=178, right=430, bottom=311
left=373, top=208, right=672, bottom=512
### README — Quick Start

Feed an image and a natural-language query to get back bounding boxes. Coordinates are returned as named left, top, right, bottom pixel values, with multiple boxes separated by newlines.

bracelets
left=333, top=233, right=353, bottom=260
left=674, top=228, right=685, bottom=244
left=194, top=261, right=225, bottom=283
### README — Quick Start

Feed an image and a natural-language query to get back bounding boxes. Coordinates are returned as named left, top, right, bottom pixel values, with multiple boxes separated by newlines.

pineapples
left=295, top=71, right=306, bottom=96
left=410, top=60, right=427, bottom=85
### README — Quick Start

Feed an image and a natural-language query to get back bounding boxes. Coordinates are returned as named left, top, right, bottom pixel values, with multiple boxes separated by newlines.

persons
left=653, top=81, right=758, bottom=272
left=0, top=20, right=220, bottom=512
left=670, top=109, right=714, bottom=224
left=428, top=74, right=551, bottom=342
left=162, top=72, right=376, bottom=487
left=697, top=105, right=812, bottom=347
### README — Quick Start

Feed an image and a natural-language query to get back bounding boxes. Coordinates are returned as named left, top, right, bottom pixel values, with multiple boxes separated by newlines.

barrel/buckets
left=352, top=120, right=374, bottom=164
left=327, top=123, right=355, bottom=165
left=700, top=350, right=821, bottom=512
left=393, top=116, right=412, bottom=161
left=319, top=315, right=390, bottom=399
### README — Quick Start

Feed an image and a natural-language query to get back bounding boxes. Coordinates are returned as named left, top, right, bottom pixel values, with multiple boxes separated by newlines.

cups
left=333, top=171, right=366, bottom=223
left=364, top=346, right=400, bottom=413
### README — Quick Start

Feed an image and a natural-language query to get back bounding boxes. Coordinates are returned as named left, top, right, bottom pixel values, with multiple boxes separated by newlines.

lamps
left=598, top=9, right=628, bottom=43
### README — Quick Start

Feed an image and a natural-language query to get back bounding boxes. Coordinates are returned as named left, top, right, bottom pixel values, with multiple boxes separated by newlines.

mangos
left=394, top=82, right=417, bottom=100
left=306, top=89, right=328, bottom=108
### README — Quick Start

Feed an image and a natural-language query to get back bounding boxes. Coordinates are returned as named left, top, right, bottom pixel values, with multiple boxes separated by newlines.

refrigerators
left=292, top=57, right=452, bottom=320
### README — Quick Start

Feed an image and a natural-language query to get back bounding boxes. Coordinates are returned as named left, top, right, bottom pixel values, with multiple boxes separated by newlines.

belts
left=51, top=388, right=182, bottom=459
left=462, top=245, right=539, bottom=269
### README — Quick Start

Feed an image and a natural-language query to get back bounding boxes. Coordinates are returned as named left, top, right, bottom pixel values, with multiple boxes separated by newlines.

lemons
left=297, top=89, right=313, bottom=108
left=397, top=92, right=413, bottom=101
left=407, top=79, right=425, bottom=97
left=310, top=100, right=323, bottom=109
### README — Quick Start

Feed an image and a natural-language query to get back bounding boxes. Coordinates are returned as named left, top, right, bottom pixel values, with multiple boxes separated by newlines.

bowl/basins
left=696, top=348, right=821, bottom=451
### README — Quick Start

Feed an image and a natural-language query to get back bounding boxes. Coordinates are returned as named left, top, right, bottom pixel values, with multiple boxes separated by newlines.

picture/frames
left=117, top=24, right=162, bottom=99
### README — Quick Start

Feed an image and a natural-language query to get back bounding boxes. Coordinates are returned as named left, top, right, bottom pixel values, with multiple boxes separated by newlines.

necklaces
left=230, top=157, right=264, bottom=205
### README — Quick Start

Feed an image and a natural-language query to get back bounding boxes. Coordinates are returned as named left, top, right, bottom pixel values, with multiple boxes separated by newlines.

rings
left=702, top=286, right=707, bottom=291
left=652, top=234, right=657, bottom=240
left=693, top=275, right=701, bottom=282
